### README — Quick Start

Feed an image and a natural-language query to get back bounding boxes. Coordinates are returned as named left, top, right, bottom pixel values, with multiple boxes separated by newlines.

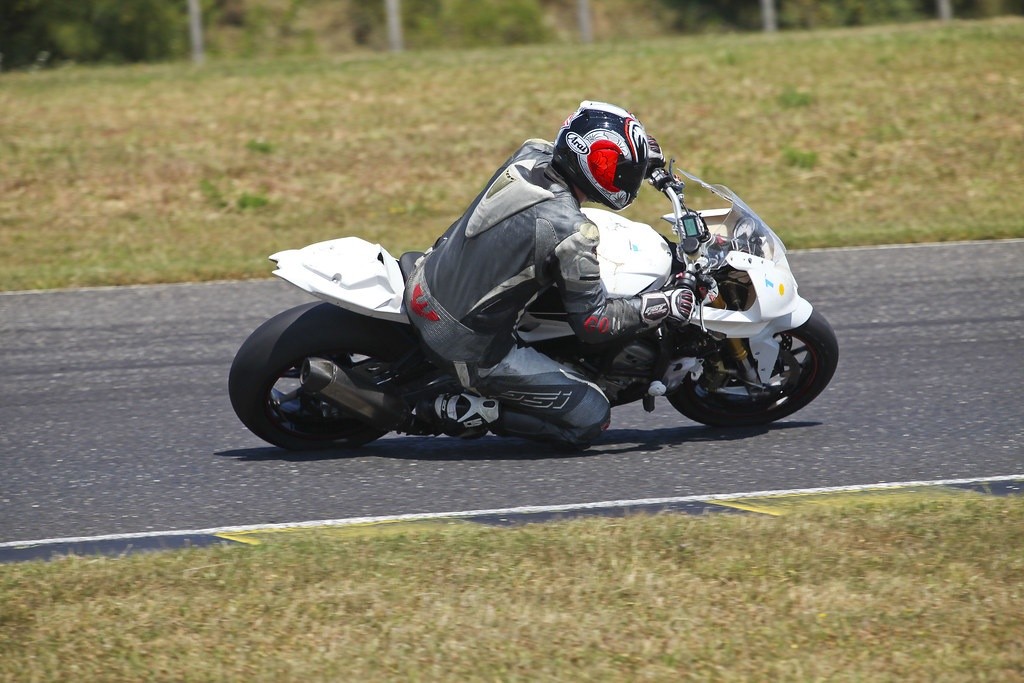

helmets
left=551, top=99, right=647, bottom=211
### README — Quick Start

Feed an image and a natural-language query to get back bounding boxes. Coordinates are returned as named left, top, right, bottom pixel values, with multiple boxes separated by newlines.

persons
left=403, top=100, right=696, bottom=451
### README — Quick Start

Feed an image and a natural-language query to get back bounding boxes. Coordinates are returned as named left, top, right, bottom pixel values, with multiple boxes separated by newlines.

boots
left=434, top=394, right=501, bottom=442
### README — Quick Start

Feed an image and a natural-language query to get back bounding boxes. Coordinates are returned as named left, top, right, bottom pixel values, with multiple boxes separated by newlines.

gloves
left=637, top=285, right=695, bottom=332
left=647, top=132, right=667, bottom=179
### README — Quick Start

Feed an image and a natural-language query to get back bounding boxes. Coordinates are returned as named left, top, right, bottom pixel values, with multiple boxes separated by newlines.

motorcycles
left=229, top=155, right=840, bottom=454
